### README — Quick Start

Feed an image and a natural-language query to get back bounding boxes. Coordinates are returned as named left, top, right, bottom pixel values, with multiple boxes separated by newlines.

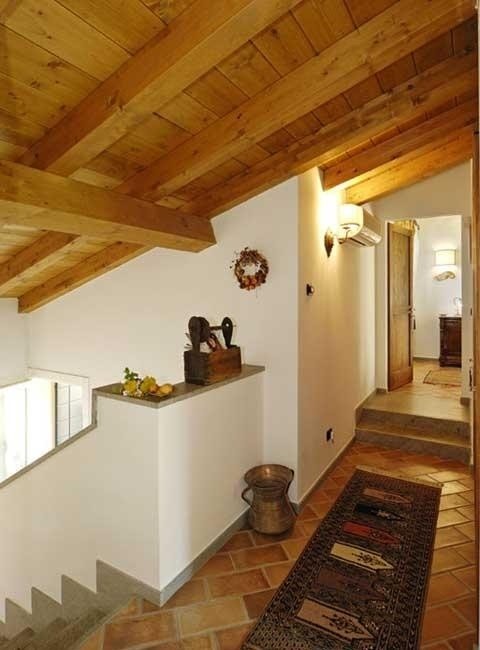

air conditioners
left=337, top=200, right=383, bottom=252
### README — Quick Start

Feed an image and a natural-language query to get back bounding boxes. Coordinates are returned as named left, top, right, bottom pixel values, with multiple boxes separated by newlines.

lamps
left=433, top=248, right=458, bottom=267
left=324, top=216, right=361, bottom=258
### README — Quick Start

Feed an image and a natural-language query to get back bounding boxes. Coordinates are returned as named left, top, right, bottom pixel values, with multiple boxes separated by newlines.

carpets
left=241, top=463, right=442, bottom=650
left=421, top=366, right=460, bottom=390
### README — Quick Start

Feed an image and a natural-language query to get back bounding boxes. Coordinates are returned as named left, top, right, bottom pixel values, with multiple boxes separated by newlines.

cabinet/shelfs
left=437, top=311, right=462, bottom=367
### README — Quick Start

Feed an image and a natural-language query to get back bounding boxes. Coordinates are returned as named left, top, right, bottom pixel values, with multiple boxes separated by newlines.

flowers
left=230, top=245, right=269, bottom=291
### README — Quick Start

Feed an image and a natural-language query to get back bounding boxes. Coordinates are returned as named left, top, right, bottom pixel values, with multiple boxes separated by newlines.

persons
left=206, top=334, right=222, bottom=351
